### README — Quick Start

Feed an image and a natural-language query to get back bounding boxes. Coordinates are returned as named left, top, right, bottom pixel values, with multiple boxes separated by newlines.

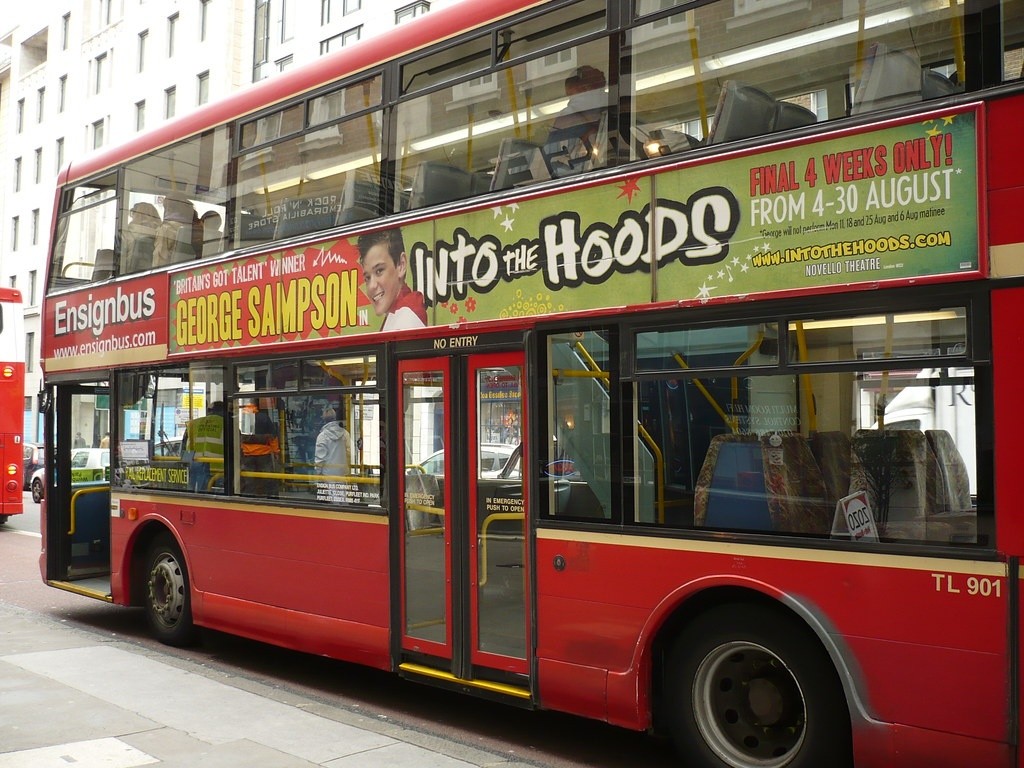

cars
left=404, top=443, right=524, bottom=479
left=153, top=441, right=186, bottom=460
left=22, top=443, right=44, bottom=489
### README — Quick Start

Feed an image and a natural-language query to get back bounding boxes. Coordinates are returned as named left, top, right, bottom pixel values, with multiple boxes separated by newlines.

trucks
left=870, top=363, right=979, bottom=500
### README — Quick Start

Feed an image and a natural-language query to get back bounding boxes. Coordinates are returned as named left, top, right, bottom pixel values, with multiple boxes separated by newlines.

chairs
left=489, top=137, right=544, bottom=192
left=923, top=68, right=955, bottom=99
left=704, top=79, right=777, bottom=146
left=273, top=196, right=333, bottom=241
left=171, top=226, right=223, bottom=263
left=216, top=213, right=276, bottom=252
left=406, top=161, right=473, bottom=209
left=693, top=418, right=977, bottom=543
left=466, top=174, right=493, bottom=197
left=333, top=178, right=405, bottom=224
left=774, top=100, right=817, bottom=132
left=850, top=41, right=922, bottom=116
left=90, top=248, right=114, bottom=282
left=555, top=479, right=572, bottom=514
left=128, top=237, right=155, bottom=271
left=356, top=473, right=443, bottom=538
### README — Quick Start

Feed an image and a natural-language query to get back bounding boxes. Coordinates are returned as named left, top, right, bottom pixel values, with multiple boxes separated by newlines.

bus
left=31, top=0, right=1024, bottom=768
left=0, top=290, right=27, bottom=523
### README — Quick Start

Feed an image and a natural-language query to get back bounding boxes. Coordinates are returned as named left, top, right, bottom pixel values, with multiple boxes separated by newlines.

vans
left=30, top=446, right=112, bottom=503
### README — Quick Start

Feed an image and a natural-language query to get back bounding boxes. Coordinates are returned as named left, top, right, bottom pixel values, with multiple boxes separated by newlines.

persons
left=357, top=227, right=428, bottom=331
left=505, top=417, right=514, bottom=443
left=99, top=432, right=110, bottom=448
left=539, top=66, right=606, bottom=176
left=179, top=402, right=244, bottom=493
left=128, top=193, right=222, bottom=273
left=313, top=408, right=354, bottom=502
left=73, top=432, right=86, bottom=448
left=241, top=373, right=285, bottom=499
